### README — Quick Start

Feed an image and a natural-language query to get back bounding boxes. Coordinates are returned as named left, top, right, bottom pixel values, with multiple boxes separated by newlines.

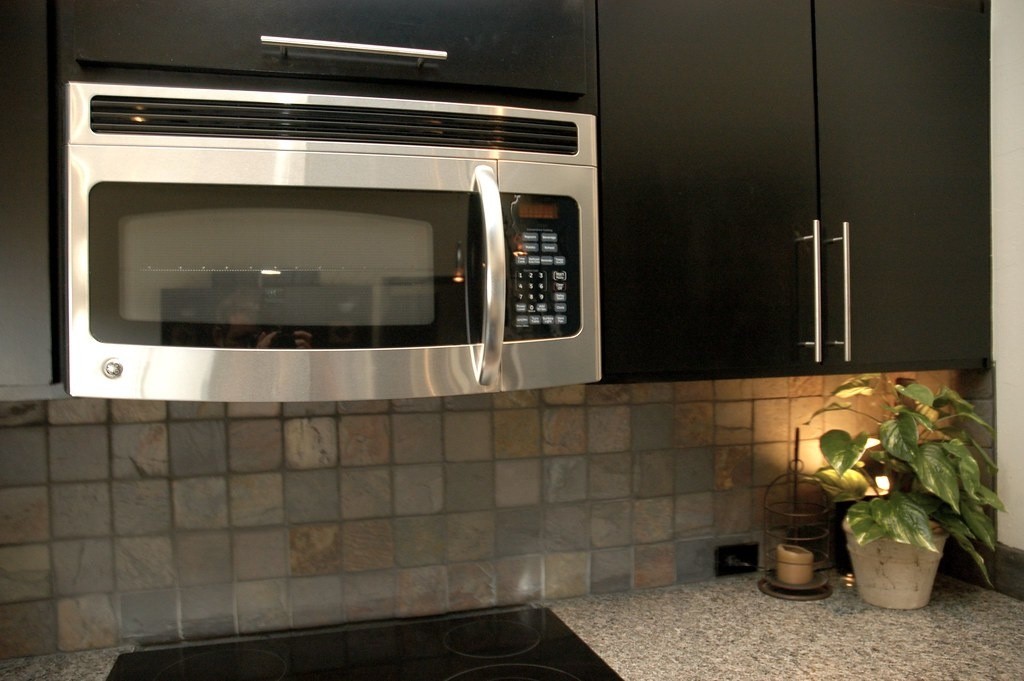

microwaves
left=59, top=82, right=600, bottom=403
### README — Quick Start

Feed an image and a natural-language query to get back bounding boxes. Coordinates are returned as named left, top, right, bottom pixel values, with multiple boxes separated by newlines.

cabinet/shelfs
left=0, top=0, right=991, bottom=402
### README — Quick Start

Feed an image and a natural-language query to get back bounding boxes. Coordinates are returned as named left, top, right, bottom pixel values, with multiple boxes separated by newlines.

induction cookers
left=105, top=609, right=622, bottom=681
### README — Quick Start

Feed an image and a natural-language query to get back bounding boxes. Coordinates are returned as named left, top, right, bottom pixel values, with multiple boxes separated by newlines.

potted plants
left=803, top=378, right=1007, bottom=610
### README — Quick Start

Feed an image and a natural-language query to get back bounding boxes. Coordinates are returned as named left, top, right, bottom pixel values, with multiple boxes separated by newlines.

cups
left=778, top=545, right=812, bottom=585
